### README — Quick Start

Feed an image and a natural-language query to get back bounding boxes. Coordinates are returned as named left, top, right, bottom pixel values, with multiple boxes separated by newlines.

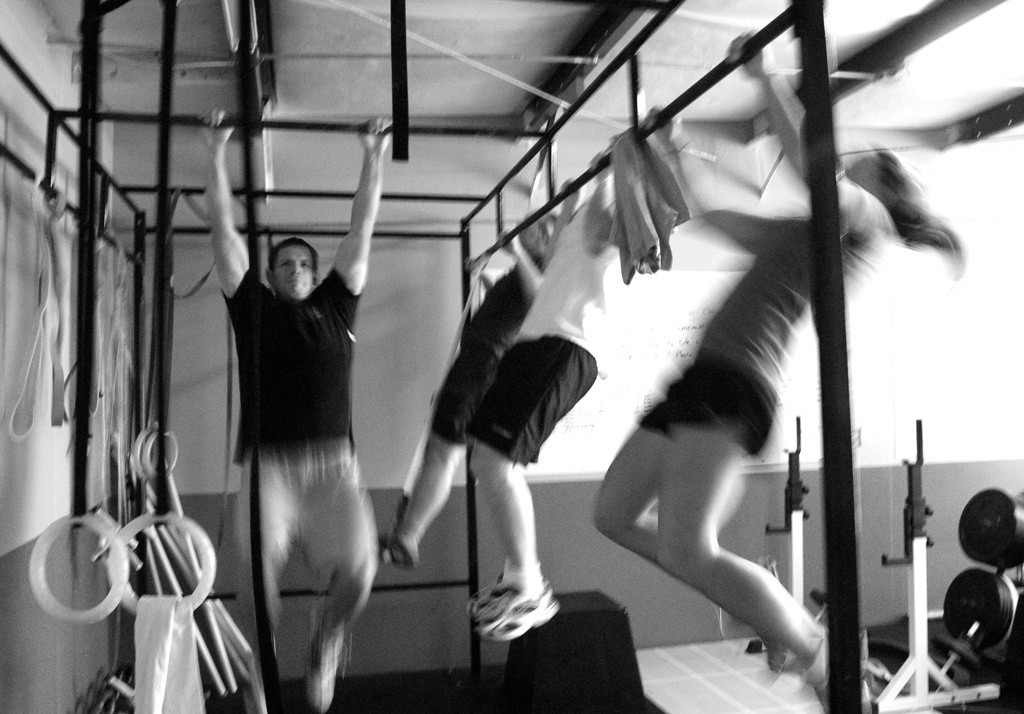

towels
left=131, top=595, right=204, bottom=714
left=611, top=127, right=694, bottom=285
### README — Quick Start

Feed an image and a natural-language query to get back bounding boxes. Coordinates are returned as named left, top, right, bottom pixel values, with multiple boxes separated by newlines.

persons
left=593, top=32, right=966, bottom=714
left=198, top=106, right=393, bottom=714
left=377, top=210, right=559, bottom=570
left=466, top=153, right=631, bottom=642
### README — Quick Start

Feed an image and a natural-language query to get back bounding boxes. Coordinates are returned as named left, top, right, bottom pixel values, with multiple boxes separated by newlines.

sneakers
left=465, top=568, right=544, bottom=621
left=478, top=578, right=561, bottom=642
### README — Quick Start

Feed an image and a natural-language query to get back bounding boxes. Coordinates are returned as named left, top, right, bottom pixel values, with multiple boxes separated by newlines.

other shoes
left=304, top=631, right=340, bottom=712
left=377, top=533, right=420, bottom=567
left=807, top=638, right=872, bottom=714
